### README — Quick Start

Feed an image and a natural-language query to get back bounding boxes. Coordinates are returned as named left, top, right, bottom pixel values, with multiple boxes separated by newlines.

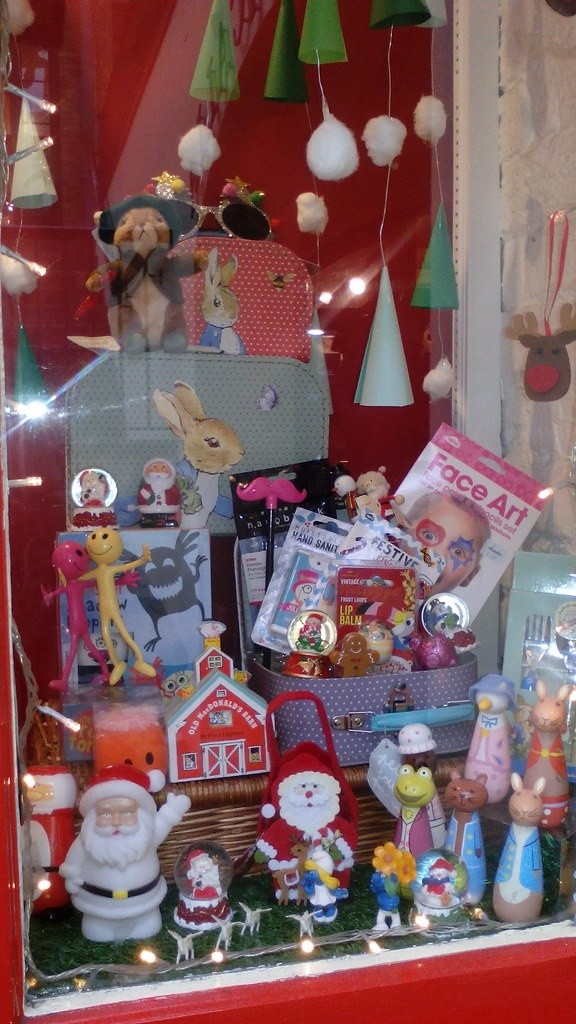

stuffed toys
left=356, top=470, right=403, bottom=528
left=85, top=195, right=208, bottom=352
left=256, top=753, right=357, bottom=900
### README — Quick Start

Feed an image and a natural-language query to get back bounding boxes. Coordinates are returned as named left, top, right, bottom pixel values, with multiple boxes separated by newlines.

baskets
left=72, top=757, right=469, bottom=884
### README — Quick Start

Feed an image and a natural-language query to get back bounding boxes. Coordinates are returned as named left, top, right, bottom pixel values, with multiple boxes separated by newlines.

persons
left=26, top=766, right=78, bottom=924
left=431, top=599, right=460, bottom=633
left=137, top=458, right=182, bottom=527
left=59, top=764, right=190, bottom=941
left=185, top=850, right=222, bottom=896
left=405, top=493, right=491, bottom=594
left=298, top=613, right=325, bottom=639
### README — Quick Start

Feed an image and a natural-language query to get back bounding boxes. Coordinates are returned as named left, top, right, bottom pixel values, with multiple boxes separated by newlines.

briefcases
left=247, top=650, right=478, bottom=766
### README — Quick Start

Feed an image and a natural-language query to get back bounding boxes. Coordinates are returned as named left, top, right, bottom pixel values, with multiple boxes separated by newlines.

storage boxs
left=61, top=685, right=166, bottom=764
left=501, top=551, right=576, bottom=784
left=247, top=640, right=483, bottom=777
